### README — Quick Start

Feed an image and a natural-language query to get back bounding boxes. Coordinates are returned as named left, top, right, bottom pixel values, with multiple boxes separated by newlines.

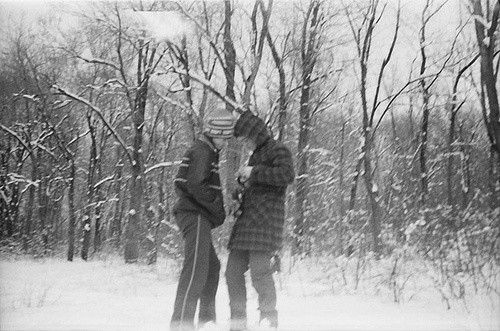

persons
left=222, top=111, right=293, bottom=329
left=169, top=108, right=236, bottom=330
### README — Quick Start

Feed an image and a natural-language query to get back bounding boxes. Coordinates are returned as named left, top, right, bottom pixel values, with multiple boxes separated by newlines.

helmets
left=204, top=108, right=235, bottom=138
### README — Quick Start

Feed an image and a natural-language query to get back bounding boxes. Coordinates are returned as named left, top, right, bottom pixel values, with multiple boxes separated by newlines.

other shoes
left=231, top=316, right=248, bottom=330
left=199, top=320, right=219, bottom=330
left=170, top=324, right=197, bottom=331
left=259, top=317, right=279, bottom=331
